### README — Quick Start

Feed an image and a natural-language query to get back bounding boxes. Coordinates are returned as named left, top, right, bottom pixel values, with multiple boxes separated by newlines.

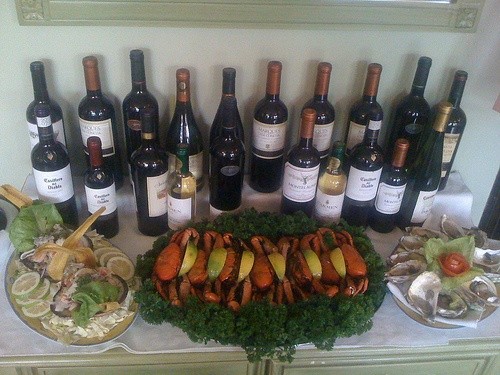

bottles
left=371, top=137, right=409, bottom=234
left=340, top=109, right=385, bottom=231
left=122, top=49, right=159, bottom=186
left=280, top=108, right=322, bottom=215
left=84, top=136, right=118, bottom=238
left=248, top=61, right=288, bottom=192
left=209, top=66, right=247, bottom=187
left=208, top=96, right=247, bottom=218
left=31, top=105, right=78, bottom=232
left=342, top=62, right=383, bottom=174
left=302, top=61, right=334, bottom=178
left=25, top=61, right=67, bottom=149
left=314, top=140, right=347, bottom=226
left=385, top=57, right=434, bottom=169
left=128, top=109, right=167, bottom=236
left=167, top=142, right=197, bottom=234
left=430, top=69, right=468, bottom=193
left=397, top=102, right=453, bottom=231
left=79, top=56, right=122, bottom=191
left=167, top=67, right=204, bottom=193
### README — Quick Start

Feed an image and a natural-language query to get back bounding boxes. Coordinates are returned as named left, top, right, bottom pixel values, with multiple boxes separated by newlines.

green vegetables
left=130, top=205, right=389, bottom=363
left=9, top=198, right=66, bottom=255
left=70, top=275, right=121, bottom=326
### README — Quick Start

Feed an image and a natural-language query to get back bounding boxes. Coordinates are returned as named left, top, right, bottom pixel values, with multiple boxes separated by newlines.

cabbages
left=424, top=234, right=485, bottom=290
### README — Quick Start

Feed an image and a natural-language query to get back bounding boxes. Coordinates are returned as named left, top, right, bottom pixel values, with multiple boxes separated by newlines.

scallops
left=20, top=224, right=129, bottom=319
left=382, top=213, right=500, bottom=322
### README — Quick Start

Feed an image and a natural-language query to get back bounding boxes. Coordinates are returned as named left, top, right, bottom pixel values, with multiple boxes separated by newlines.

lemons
left=301, top=248, right=322, bottom=279
left=11, top=272, right=56, bottom=319
left=238, top=250, right=255, bottom=282
left=329, top=247, right=347, bottom=278
left=207, top=247, right=228, bottom=282
left=178, top=240, right=197, bottom=276
left=94, top=246, right=135, bottom=282
left=267, top=252, right=286, bottom=281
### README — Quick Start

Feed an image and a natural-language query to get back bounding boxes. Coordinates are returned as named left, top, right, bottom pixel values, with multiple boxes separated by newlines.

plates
left=5, top=231, right=142, bottom=349
left=387, top=229, right=500, bottom=329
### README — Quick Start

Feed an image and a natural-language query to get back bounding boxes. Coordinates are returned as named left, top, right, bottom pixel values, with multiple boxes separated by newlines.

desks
left=0, top=225, right=499, bottom=375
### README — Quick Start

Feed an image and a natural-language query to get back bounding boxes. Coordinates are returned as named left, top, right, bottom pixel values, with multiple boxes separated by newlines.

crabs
left=151, top=229, right=369, bottom=308
